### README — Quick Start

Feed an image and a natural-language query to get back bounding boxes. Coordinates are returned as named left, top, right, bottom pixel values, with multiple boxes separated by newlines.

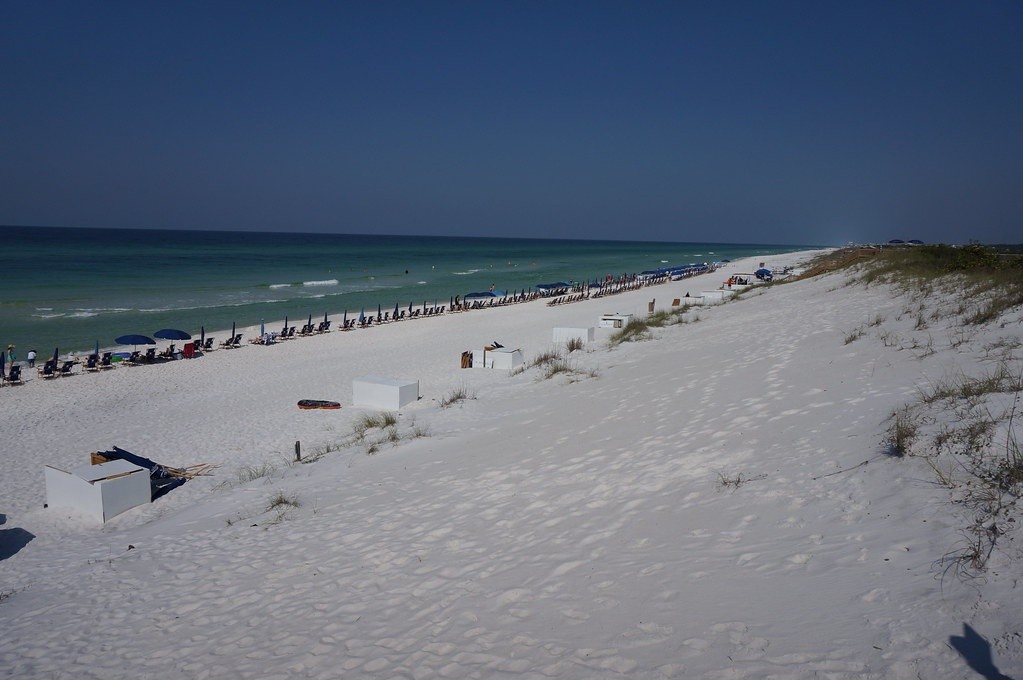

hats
left=8, top=344, right=15, bottom=349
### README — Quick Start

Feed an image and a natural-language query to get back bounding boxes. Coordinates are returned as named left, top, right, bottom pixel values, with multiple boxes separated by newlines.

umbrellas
left=285, top=311, right=327, bottom=330
left=0, top=351, right=5, bottom=378
left=52, top=347, right=59, bottom=370
left=536, top=263, right=705, bottom=292
left=200, top=318, right=265, bottom=346
left=154, top=329, right=191, bottom=345
left=95, top=340, right=98, bottom=355
left=344, top=302, right=399, bottom=324
left=464, top=286, right=531, bottom=303
left=409, top=295, right=453, bottom=314
left=114, top=334, right=157, bottom=352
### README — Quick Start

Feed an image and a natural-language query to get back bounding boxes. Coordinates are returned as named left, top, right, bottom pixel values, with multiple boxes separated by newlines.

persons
left=161, top=345, right=175, bottom=356
left=27, top=349, right=37, bottom=368
left=8, top=344, right=16, bottom=369
left=455, top=295, right=460, bottom=309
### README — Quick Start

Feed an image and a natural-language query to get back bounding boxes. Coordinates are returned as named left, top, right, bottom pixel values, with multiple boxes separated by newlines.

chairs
left=451, top=287, right=568, bottom=311
left=250, top=306, right=331, bottom=344
left=0, top=333, right=243, bottom=384
left=337, top=305, right=445, bottom=331
left=550, top=274, right=670, bottom=305
